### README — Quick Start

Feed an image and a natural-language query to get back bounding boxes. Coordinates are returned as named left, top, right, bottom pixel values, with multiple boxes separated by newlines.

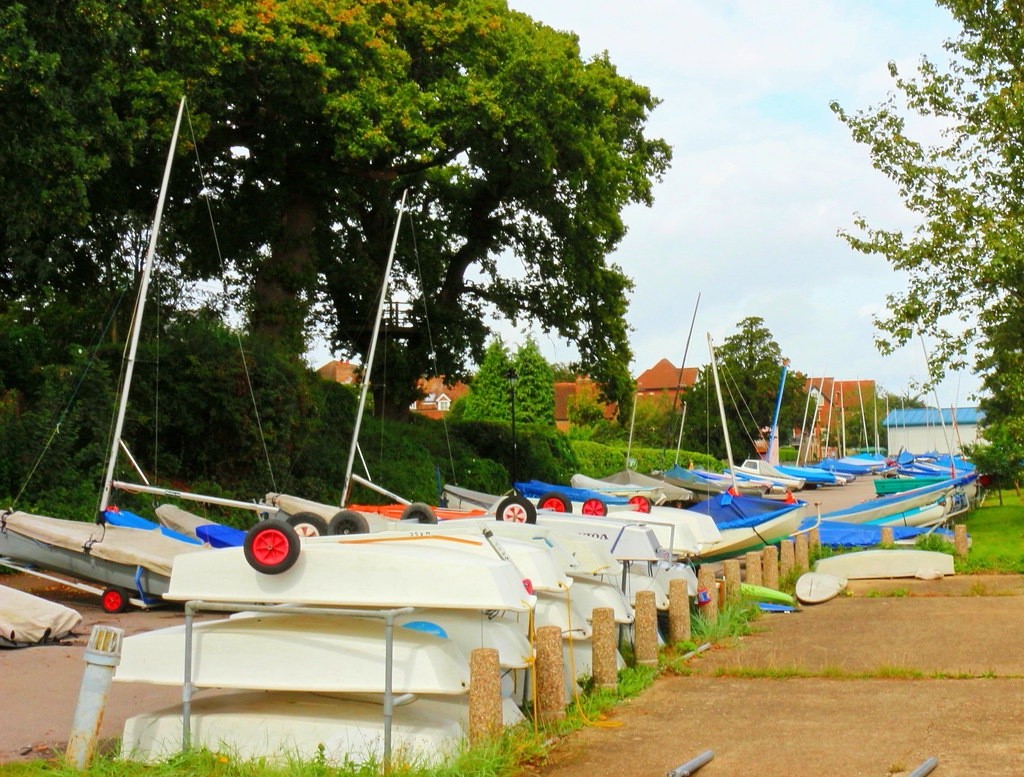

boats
left=0, top=585, right=83, bottom=649
left=812, top=549, right=956, bottom=582
left=0, top=96, right=983, bottom=766
